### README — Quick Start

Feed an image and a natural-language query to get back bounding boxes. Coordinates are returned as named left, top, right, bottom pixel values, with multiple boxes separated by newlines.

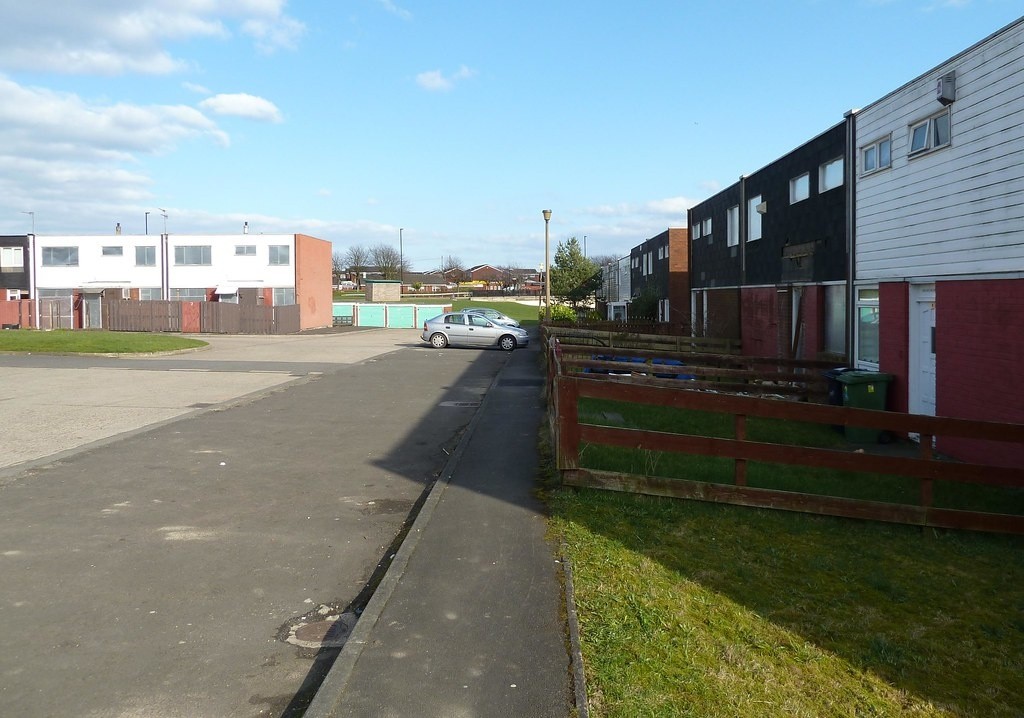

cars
left=420, top=312, right=529, bottom=352
left=460, top=307, right=520, bottom=328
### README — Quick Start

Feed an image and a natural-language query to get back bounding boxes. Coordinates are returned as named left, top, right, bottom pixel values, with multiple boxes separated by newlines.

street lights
left=399, top=228, right=406, bottom=285
left=542, top=208, right=551, bottom=327
left=584, top=235, right=587, bottom=258
left=145, top=211, right=150, bottom=234
